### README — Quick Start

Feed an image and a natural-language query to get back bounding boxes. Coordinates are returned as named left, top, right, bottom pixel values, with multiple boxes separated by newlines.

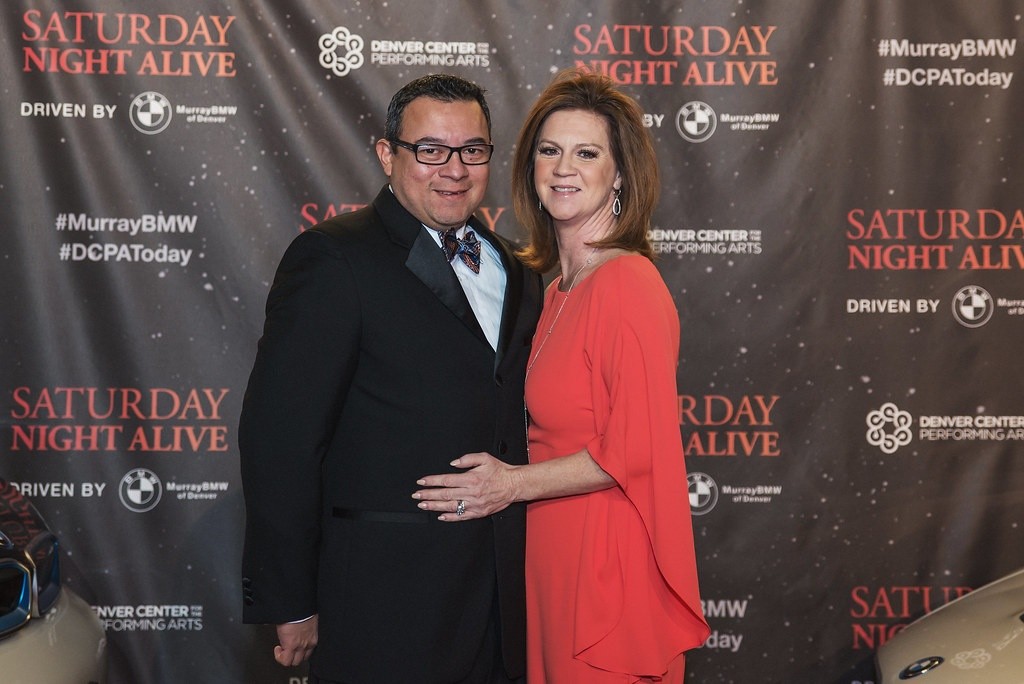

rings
left=456, top=500, right=466, bottom=515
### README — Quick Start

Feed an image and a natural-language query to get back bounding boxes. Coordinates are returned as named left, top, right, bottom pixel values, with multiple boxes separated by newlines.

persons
left=238, top=74, right=542, bottom=684
left=412, top=75, right=711, bottom=684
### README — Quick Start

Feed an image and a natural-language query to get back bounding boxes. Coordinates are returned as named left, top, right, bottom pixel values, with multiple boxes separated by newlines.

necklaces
left=523, top=229, right=613, bottom=465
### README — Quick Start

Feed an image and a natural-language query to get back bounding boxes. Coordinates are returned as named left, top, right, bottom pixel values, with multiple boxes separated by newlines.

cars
left=843, top=568, right=1024, bottom=684
left=1, top=479, right=112, bottom=684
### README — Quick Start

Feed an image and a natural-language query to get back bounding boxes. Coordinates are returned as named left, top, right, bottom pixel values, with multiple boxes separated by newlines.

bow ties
left=439, top=227, right=481, bottom=274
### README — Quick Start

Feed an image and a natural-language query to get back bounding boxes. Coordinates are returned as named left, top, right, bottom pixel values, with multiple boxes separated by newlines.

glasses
left=389, top=138, right=494, bottom=165
